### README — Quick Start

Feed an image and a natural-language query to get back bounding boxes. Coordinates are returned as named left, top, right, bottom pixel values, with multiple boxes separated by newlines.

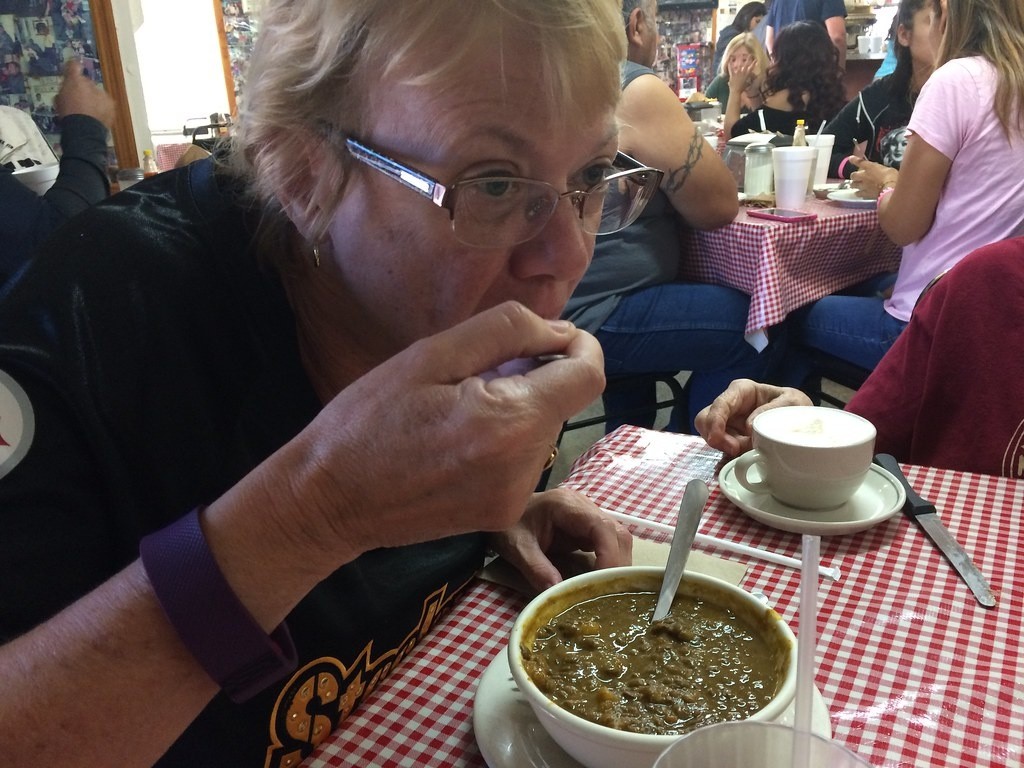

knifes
left=873, top=454, right=997, bottom=607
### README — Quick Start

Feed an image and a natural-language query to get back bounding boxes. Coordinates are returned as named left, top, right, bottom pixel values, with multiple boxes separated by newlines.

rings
left=542, top=445, right=558, bottom=471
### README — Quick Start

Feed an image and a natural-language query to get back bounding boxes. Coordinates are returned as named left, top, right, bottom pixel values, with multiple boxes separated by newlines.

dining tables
left=291, top=421, right=1024, bottom=768
left=681, top=176, right=902, bottom=355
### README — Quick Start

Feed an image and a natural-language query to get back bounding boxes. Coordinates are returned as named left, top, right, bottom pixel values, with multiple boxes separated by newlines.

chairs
left=801, top=235, right=1024, bottom=479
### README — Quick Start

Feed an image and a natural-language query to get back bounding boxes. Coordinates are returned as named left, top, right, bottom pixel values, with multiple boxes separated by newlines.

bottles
left=744, top=143, right=775, bottom=196
left=143, top=150, right=158, bottom=179
left=791, top=119, right=806, bottom=146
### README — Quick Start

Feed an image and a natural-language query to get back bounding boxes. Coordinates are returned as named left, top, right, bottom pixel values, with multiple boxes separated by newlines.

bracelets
left=877, top=186, right=895, bottom=210
left=138, top=508, right=299, bottom=705
left=747, top=93, right=760, bottom=98
left=837, top=157, right=850, bottom=179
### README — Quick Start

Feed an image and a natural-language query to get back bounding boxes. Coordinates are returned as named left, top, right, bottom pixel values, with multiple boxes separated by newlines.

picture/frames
left=212, top=0, right=274, bottom=123
left=0, top=0, right=141, bottom=172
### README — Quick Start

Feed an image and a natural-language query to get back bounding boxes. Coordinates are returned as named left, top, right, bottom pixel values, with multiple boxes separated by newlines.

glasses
left=312, top=118, right=665, bottom=250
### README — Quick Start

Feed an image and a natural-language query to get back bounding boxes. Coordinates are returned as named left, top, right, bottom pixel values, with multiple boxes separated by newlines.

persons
left=0, top=0, right=116, bottom=163
left=560, top=0, right=773, bottom=435
left=785, top=0, right=1024, bottom=407
left=0, top=0, right=632, bottom=768
left=706, top=33, right=764, bottom=114
left=694, top=238, right=1024, bottom=480
left=724, top=20, right=847, bottom=139
left=654, top=8, right=713, bottom=94
left=713, top=0, right=847, bottom=78
left=0, top=61, right=115, bottom=285
left=222, top=0, right=260, bottom=105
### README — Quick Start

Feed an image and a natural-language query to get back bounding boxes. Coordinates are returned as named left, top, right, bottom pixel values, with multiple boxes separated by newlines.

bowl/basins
left=12, top=162, right=59, bottom=196
left=507, top=565, right=799, bottom=768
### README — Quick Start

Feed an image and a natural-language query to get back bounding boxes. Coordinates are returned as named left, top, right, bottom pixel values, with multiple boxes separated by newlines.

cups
left=654, top=719, right=874, bottom=768
left=870, top=36, right=882, bottom=53
left=857, top=36, right=870, bottom=54
left=805, top=134, right=836, bottom=184
left=734, top=405, right=877, bottom=510
left=772, top=145, right=814, bottom=210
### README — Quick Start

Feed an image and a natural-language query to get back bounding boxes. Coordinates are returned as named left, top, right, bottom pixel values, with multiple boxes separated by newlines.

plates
left=827, top=188, right=877, bottom=210
left=718, top=456, right=907, bottom=535
left=813, top=183, right=844, bottom=199
left=472, top=643, right=832, bottom=768
